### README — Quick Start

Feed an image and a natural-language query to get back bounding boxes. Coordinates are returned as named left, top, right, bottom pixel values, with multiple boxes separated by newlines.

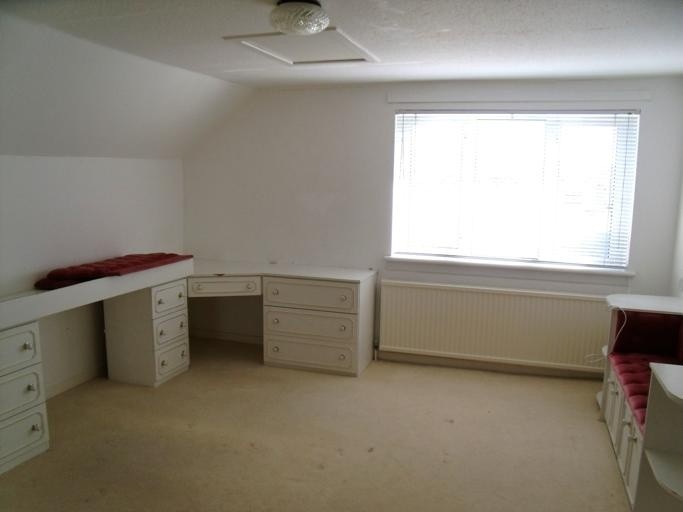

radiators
left=379, top=280, right=613, bottom=374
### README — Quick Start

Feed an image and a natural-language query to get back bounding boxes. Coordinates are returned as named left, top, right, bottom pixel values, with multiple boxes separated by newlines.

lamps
left=268, top=0, right=329, bottom=37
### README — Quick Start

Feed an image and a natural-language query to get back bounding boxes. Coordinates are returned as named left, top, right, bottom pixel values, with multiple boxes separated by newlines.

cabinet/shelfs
left=187, top=275, right=262, bottom=297
left=594, top=293, right=683, bottom=512
left=0, top=319, right=50, bottom=475
left=102, top=278, right=191, bottom=388
left=262, top=275, right=374, bottom=377
